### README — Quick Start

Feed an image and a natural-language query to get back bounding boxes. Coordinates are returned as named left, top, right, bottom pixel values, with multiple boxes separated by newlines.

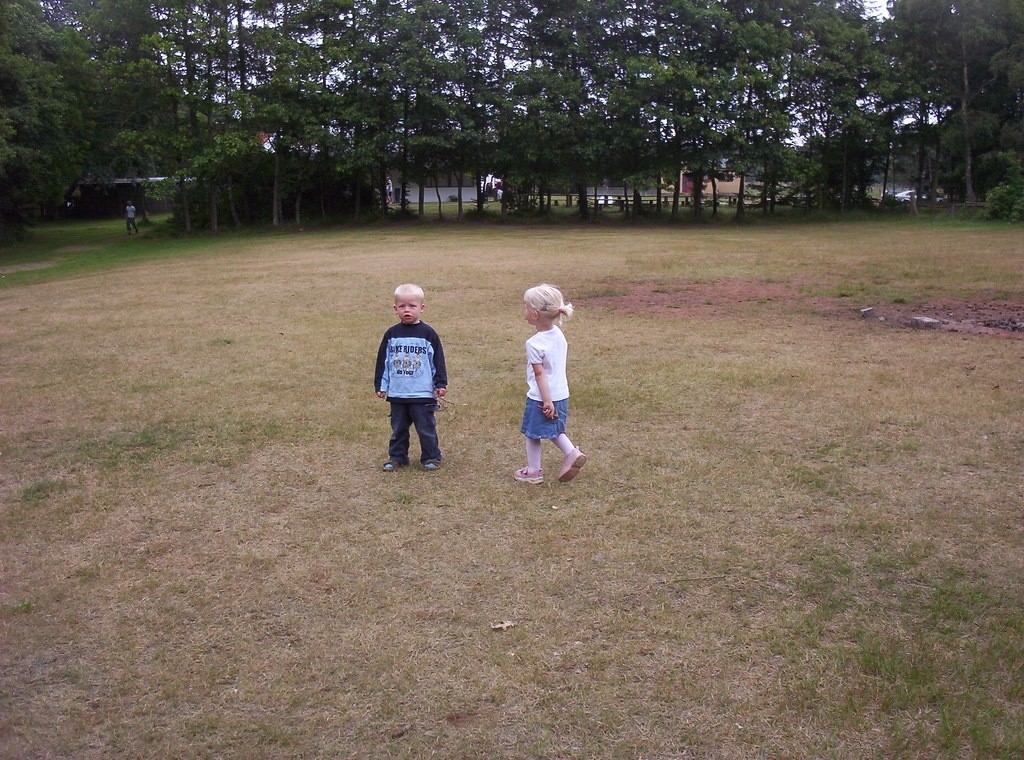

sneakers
left=514, top=466, right=544, bottom=485
left=558, top=446, right=587, bottom=482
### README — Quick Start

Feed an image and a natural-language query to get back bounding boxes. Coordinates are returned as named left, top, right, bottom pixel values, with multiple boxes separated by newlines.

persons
left=373, top=284, right=449, bottom=471
left=386, top=176, right=393, bottom=205
left=514, top=284, right=587, bottom=483
left=127, top=200, right=139, bottom=235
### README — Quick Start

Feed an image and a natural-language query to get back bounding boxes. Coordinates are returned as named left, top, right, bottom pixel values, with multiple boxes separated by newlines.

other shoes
left=424, top=459, right=441, bottom=469
left=383, top=461, right=410, bottom=472
left=136, top=229, right=138, bottom=233
left=128, top=232, right=131, bottom=234
